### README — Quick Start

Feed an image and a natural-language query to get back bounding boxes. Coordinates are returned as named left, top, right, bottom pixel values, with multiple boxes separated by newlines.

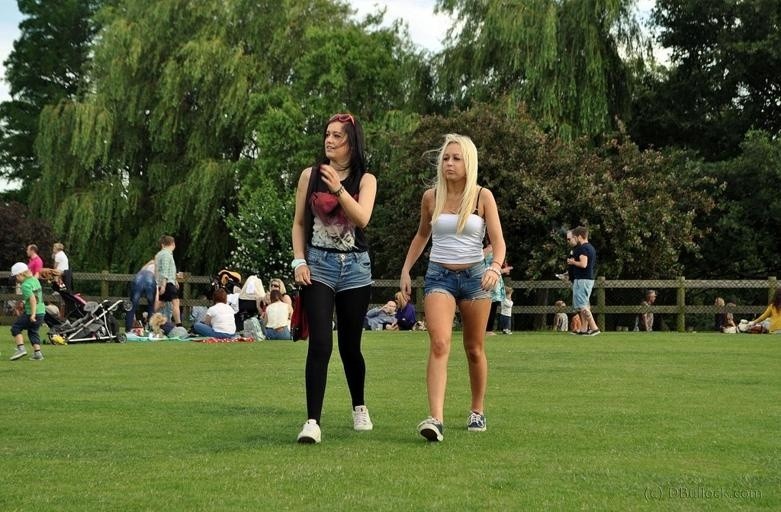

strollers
left=47, top=283, right=91, bottom=319
left=42, top=296, right=142, bottom=344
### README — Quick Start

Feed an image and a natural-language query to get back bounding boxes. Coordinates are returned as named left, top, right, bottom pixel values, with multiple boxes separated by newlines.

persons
left=633, top=290, right=657, bottom=332
left=11, top=235, right=515, bottom=365
left=552, top=227, right=601, bottom=337
left=292, top=113, right=378, bottom=445
left=714, top=285, right=781, bottom=334
left=400, top=133, right=507, bottom=442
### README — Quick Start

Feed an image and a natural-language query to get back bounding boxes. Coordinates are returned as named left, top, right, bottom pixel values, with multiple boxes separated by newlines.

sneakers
left=584, top=328, right=600, bottom=336
left=297, top=419, right=321, bottom=443
left=352, top=405, right=373, bottom=430
left=468, top=412, right=486, bottom=431
left=10, top=349, right=27, bottom=360
left=33, top=351, right=42, bottom=360
left=419, top=416, right=444, bottom=442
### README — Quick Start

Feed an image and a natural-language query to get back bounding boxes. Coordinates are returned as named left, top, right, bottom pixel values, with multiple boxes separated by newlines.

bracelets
left=331, top=185, right=344, bottom=198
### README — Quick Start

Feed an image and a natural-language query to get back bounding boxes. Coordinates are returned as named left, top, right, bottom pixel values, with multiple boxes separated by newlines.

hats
left=11, top=262, right=29, bottom=276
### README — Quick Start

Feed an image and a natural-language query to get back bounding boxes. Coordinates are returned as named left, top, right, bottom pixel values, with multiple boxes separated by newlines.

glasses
left=271, top=284, right=279, bottom=287
left=329, top=114, right=356, bottom=124
